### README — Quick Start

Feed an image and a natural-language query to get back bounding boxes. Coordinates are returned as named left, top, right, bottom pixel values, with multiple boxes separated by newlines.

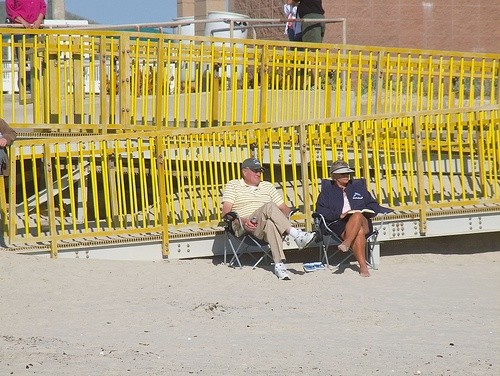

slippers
left=303, top=260, right=325, bottom=271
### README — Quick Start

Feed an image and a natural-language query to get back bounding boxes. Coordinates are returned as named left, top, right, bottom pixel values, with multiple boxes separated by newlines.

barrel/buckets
left=172, top=17, right=196, bottom=82
left=201, top=11, right=251, bottom=81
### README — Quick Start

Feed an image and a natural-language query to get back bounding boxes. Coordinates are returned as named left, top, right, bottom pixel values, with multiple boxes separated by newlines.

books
left=340, top=209, right=375, bottom=219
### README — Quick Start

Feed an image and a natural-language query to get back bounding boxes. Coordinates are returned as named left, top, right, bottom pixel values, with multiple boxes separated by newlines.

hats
left=241, top=158, right=265, bottom=172
left=330, top=160, right=354, bottom=173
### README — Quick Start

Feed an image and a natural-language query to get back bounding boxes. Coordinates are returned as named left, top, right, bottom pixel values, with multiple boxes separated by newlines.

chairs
left=222, top=208, right=300, bottom=270
left=310, top=178, right=394, bottom=272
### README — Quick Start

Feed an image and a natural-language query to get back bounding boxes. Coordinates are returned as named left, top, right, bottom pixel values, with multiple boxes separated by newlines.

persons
left=6, top=0, right=47, bottom=94
left=283, top=0, right=325, bottom=90
left=221, top=158, right=317, bottom=280
left=0, top=118, right=17, bottom=175
left=316, top=160, right=379, bottom=277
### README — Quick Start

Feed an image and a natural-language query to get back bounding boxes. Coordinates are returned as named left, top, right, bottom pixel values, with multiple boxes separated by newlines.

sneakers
left=295, top=232, right=317, bottom=252
left=275, top=263, right=291, bottom=281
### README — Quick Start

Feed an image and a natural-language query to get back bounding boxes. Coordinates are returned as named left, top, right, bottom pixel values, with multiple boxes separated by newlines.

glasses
left=251, top=170, right=261, bottom=174
